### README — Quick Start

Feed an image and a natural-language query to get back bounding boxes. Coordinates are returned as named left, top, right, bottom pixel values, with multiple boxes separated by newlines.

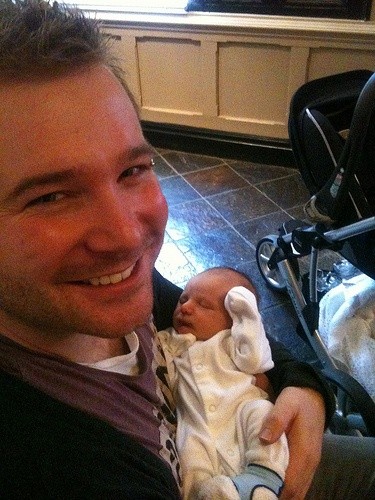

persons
left=159, top=267, right=291, bottom=500
left=0, top=0, right=375, bottom=500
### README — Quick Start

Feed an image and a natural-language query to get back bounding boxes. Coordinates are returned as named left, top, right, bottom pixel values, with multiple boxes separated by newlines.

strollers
left=256, top=69, right=375, bottom=436
